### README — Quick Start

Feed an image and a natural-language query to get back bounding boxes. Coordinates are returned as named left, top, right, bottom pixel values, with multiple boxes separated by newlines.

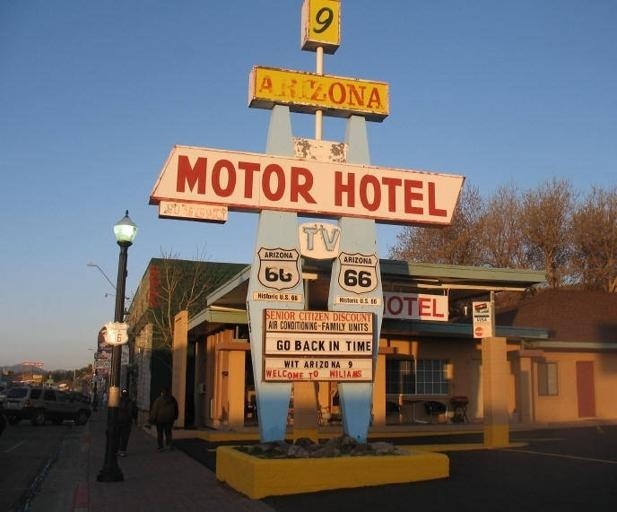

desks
left=402, top=395, right=426, bottom=426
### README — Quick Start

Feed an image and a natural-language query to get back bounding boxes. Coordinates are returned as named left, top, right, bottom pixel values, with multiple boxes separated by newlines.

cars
left=0, top=389, right=8, bottom=404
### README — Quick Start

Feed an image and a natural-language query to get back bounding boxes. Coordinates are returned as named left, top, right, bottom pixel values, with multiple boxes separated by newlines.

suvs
left=4, top=386, right=91, bottom=426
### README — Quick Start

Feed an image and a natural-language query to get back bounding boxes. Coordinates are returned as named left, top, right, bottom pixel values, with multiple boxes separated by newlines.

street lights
left=96, top=210, right=138, bottom=483
left=88, top=263, right=117, bottom=292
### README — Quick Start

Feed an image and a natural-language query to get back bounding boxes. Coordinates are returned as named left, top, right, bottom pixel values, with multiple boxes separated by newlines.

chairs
left=383, top=398, right=402, bottom=425
left=447, top=394, right=475, bottom=425
left=423, top=398, right=447, bottom=425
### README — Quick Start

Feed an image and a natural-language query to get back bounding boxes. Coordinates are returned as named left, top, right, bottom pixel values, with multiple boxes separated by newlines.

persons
left=146, top=388, right=179, bottom=450
left=116, top=389, right=137, bottom=457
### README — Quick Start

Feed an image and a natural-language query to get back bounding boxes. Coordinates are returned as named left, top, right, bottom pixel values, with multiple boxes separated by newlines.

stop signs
left=474, top=326, right=492, bottom=337
